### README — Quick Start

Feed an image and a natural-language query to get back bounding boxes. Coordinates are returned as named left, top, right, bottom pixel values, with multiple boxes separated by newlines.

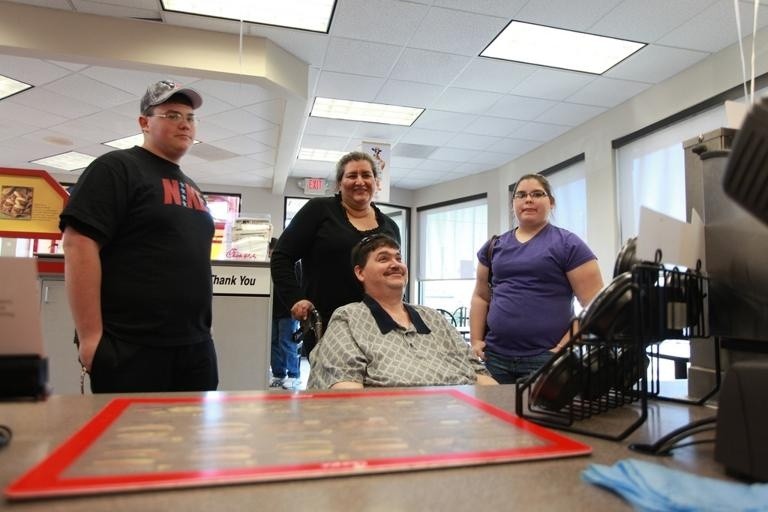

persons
left=467, top=174, right=606, bottom=383
left=306, top=230, right=502, bottom=391
left=269, top=150, right=401, bottom=366
left=267, top=235, right=302, bottom=390
left=59, top=80, right=219, bottom=395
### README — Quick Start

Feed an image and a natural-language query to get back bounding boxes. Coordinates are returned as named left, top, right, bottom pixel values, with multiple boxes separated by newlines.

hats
left=269, top=237, right=279, bottom=249
left=141, top=80, right=202, bottom=116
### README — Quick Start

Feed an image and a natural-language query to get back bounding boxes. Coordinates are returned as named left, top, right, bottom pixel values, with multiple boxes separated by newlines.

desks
left=0, top=383, right=739, bottom=512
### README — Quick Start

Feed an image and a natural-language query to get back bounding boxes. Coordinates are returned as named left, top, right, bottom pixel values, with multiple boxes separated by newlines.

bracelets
left=555, top=340, right=565, bottom=349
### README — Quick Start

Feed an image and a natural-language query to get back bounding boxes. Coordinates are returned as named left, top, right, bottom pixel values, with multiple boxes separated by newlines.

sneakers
left=269, top=377, right=301, bottom=389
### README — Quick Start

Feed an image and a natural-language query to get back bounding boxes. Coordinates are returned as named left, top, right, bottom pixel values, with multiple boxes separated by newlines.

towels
left=580, top=458, right=768, bottom=512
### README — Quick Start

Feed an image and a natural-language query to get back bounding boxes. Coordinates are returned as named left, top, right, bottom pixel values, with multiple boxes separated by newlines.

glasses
left=359, top=233, right=401, bottom=256
left=151, top=113, right=195, bottom=123
left=515, top=192, right=548, bottom=198
